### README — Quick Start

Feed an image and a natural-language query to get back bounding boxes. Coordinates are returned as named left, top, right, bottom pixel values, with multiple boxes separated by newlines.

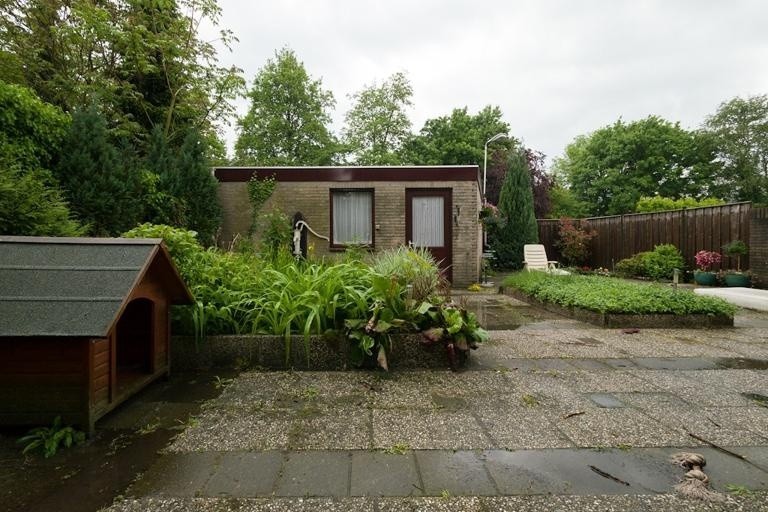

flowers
left=691, top=249, right=722, bottom=272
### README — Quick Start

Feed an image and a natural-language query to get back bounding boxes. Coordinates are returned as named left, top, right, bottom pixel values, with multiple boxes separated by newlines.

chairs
left=520, top=242, right=571, bottom=277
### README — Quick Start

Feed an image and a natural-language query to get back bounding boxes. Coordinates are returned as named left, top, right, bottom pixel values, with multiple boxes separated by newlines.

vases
left=694, top=272, right=714, bottom=283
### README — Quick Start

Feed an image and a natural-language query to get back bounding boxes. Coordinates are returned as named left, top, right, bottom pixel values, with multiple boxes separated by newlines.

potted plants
left=722, top=238, right=751, bottom=287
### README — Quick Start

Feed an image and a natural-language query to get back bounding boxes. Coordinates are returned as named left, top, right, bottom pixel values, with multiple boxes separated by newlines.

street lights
left=483, top=132, right=507, bottom=247
